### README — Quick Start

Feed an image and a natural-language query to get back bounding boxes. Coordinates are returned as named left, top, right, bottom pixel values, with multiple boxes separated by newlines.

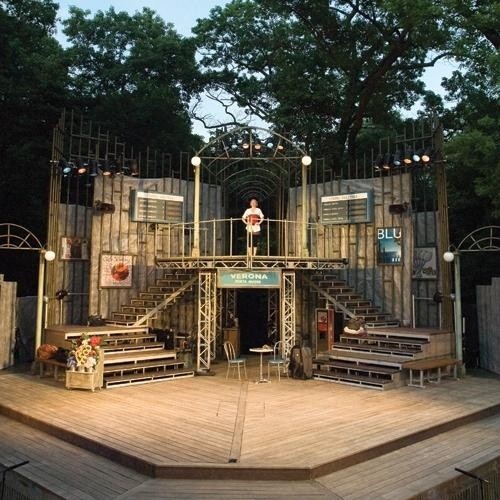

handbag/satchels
left=38, top=344, right=58, bottom=359
left=54, top=347, right=69, bottom=362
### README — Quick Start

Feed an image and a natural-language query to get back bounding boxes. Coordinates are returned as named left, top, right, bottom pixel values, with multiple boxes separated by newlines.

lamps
left=210, top=138, right=288, bottom=153
left=443, top=243, right=459, bottom=263
left=301, top=151, right=312, bottom=166
left=374, top=146, right=435, bottom=171
left=191, top=149, right=202, bottom=165
left=61, top=151, right=140, bottom=178
left=45, top=250, right=55, bottom=262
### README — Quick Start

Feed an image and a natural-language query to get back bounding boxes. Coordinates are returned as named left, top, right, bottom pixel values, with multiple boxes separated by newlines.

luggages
left=291, top=340, right=313, bottom=379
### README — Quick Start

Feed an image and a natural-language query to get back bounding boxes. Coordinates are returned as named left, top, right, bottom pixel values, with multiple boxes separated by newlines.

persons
left=241, top=199, right=264, bottom=256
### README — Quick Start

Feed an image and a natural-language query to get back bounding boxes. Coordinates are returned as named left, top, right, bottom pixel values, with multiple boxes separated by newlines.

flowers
left=68, top=332, right=102, bottom=371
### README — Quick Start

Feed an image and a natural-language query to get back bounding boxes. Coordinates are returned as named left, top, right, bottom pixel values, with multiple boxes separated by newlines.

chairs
left=222, top=340, right=247, bottom=381
left=267, top=340, right=294, bottom=382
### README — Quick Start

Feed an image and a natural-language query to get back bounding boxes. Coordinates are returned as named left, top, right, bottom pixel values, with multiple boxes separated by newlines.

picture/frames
left=59, top=235, right=91, bottom=263
left=98, top=251, right=135, bottom=291
left=410, top=245, right=439, bottom=282
left=373, top=226, right=404, bottom=267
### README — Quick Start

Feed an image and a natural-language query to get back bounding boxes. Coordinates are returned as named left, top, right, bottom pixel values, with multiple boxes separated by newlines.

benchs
left=36, top=353, right=68, bottom=382
left=403, top=356, right=464, bottom=387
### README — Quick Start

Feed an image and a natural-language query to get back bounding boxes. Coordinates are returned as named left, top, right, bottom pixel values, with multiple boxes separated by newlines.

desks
left=248, top=348, right=277, bottom=385
